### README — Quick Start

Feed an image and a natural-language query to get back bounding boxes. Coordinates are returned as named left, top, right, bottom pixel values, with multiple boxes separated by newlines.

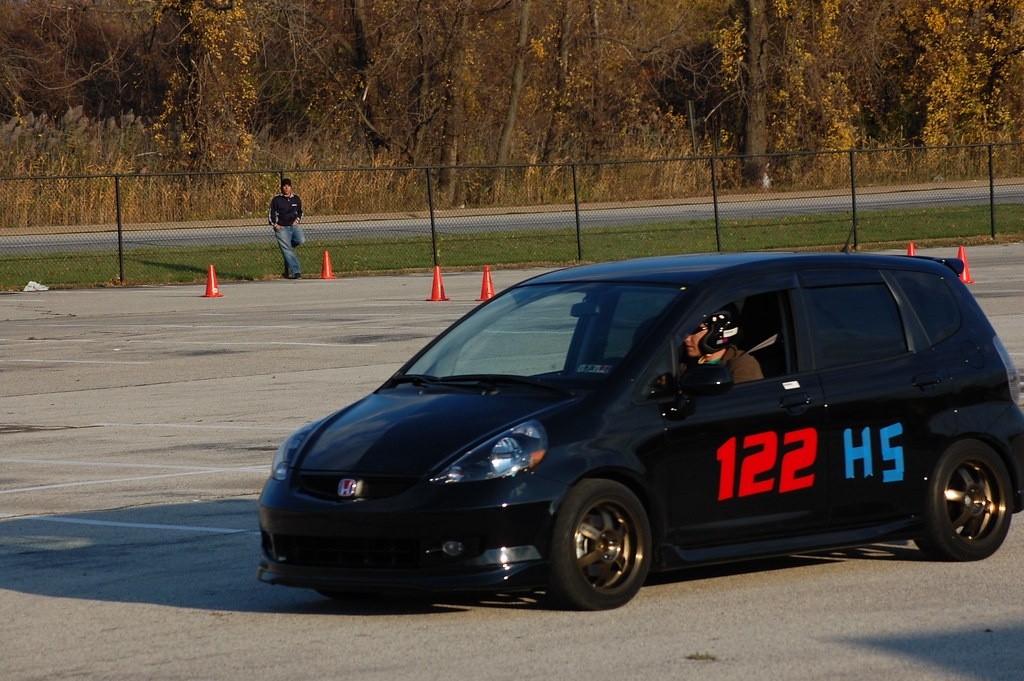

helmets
left=698, top=302, right=744, bottom=356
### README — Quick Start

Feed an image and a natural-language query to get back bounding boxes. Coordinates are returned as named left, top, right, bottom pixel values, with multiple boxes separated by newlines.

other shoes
left=294, top=273, right=301, bottom=279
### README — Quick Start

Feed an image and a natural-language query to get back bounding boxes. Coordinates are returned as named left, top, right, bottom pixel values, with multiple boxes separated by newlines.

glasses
left=687, top=326, right=708, bottom=335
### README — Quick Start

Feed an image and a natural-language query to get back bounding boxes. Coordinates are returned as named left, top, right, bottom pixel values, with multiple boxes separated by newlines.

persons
left=267, top=178, right=306, bottom=279
left=679, top=309, right=764, bottom=383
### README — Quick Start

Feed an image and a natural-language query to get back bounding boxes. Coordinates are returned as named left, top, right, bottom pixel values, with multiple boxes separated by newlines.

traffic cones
left=955, top=246, right=975, bottom=285
left=474, top=265, right=496, bottom=302
left=425, top=265, right=450, bottom=303
left=318, top=250, right=336, bottom=279
left=201, top=264, right=225, bottom=298
left=907, top=243, right=916, bottom=257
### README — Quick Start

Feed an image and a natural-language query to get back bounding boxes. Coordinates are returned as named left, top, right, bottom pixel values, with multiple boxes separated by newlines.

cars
left=257, top=246, right=1023, bottom=614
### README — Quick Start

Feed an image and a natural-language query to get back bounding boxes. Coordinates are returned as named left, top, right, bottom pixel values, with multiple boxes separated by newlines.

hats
left=281, top=178, right=291, bottom=186
